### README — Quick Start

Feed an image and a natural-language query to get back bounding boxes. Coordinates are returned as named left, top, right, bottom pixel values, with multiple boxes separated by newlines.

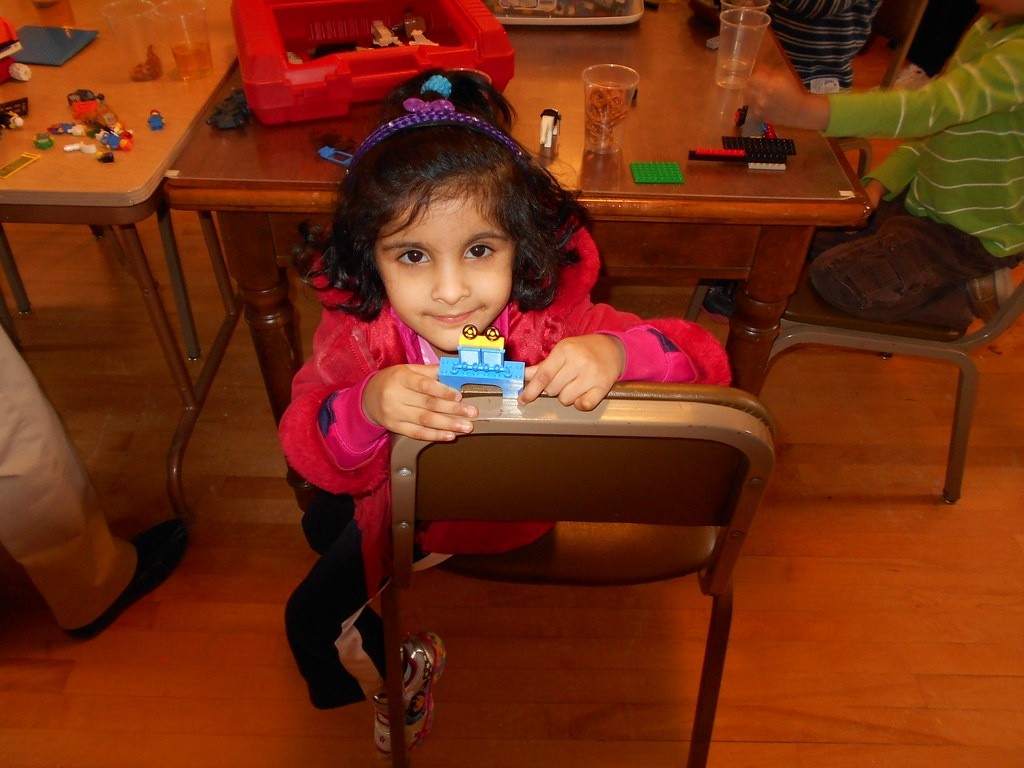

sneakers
left=374, top=632, right=446, bottom=756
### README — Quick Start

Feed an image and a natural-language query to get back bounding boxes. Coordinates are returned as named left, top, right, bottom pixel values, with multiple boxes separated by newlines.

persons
left=0, top=324, right=188, bottom=638
left=689, top=0, right=881, bottom=93
left=284, top=69, right=733, bottom=757
left=747, top=0, right=1023, bottom=326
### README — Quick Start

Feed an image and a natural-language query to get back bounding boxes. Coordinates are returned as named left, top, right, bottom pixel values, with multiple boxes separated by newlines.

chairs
left=380, top=377, right=771, bottom=765
left=752, top=282, right=1021, bottom=503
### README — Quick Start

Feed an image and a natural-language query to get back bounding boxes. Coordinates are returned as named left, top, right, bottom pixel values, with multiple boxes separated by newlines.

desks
left=0, top=0, right=294, bottom=518
left=163, top=1, right=874, bottom=404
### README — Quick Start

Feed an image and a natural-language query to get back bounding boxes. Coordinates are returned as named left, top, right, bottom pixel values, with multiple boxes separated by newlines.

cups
left=715, top=9, right=771, bottom=90
left=719, top=0, right=770, bottom=19
left=154, top=0, right=214, bottom=82
left=582, top=63, right=639, bottom=156
left=30, top=0, right=75, bottom=30
left=102, top=0, right=162, bottom=84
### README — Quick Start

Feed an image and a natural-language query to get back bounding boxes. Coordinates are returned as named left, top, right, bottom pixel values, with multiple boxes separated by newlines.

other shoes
left=701, top=290, right=733, bottom=325
left=65, top=518, right=189, bottom=640
left=966, top=265, right=1012, bottom=324
